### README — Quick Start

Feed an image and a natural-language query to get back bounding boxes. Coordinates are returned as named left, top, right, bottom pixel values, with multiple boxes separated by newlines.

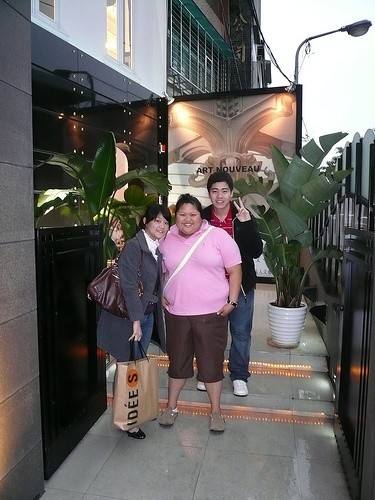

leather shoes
left=126, top=428, right=146, bottom=439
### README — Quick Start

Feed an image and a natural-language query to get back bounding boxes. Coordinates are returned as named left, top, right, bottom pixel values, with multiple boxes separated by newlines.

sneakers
left=158, top=407, right=178, bottom=426
left=209, top=413, right=226, bottom=431
left=196, top=381, right=207, bottom=391
left=232, top=380, right=248, bottom=396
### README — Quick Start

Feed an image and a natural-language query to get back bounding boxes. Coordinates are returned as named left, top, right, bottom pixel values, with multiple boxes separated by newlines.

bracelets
left=228, top=301, right=238, bottom=308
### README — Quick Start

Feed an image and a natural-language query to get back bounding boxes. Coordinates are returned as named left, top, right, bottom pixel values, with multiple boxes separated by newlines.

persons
left=198, top=171, right=263, bottom=396
left=97, top=204, right=171, bottom=439
left=158, top=194, right=242, bottom=432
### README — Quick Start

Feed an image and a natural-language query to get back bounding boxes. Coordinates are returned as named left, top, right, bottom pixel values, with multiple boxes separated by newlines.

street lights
left=293, top=19, right=372, bottom=85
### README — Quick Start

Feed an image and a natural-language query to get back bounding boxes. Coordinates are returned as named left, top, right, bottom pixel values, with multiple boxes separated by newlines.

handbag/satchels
left=111, top=336, right=159, bottom=431
left=87, top=242, right=144, bottom=318
